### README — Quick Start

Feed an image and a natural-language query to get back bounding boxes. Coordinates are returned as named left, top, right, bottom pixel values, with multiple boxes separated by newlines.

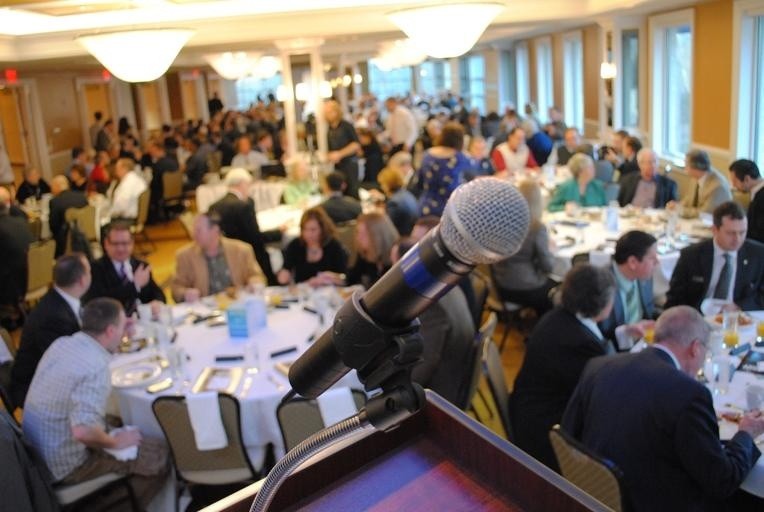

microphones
left=288, top=176, right=530, bottom=399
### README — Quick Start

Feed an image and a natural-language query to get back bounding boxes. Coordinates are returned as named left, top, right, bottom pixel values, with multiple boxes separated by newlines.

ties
left=626, top=284, right=639, bottom=325
left=119, top=262, right=130, bottom=287
left=713, top=253, right=733, bottom=300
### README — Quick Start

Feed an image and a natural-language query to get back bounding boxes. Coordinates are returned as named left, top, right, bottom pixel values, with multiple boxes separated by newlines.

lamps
left=385, top=4, right=503, bottom=62
left=77, top=27, right=194, bottom=86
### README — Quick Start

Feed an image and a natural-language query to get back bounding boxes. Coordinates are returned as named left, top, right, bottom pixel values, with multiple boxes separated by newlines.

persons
left=2, top=90, right=763, bottom=509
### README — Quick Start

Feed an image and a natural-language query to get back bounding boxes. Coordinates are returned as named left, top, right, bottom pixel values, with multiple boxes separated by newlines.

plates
left=112, top=360, right=161, bottom=389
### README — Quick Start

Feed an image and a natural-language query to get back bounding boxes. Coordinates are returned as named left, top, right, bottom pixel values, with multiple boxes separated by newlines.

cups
left=722, top=311, right=738, bottom=349
left=744, top=383, right=763, bottom=445
left=711, top=358, right=729, bottom=395
left=144, top=323, right=160, bottom=350
left=166, top=345, right=183, bottom=379
